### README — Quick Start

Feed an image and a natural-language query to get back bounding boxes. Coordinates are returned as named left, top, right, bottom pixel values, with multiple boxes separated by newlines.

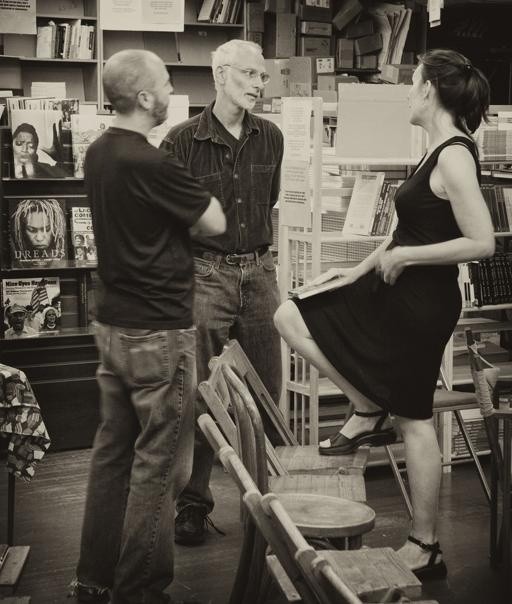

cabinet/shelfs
left=0, top=0, right=100, bottom=456
left=100, top=0, right=250, bottom=124
left=278, top=85, right=512, bottom=469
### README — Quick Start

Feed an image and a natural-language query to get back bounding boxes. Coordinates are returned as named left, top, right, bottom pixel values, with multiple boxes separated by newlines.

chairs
left=222, top=445, right=427, bottom=602
left=198, top=356, right=373, bottom=504
left=360, top=364, right=494, bottom=535
left=212, top=339, right=374, bottom=475
left=462, top=326, right=511, bottom=576
left=193, top=406, right=377, bottom=603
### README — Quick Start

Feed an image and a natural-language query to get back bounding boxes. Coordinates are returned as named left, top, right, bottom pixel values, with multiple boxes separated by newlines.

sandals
left=319, top=410, right=396, bottom=455
left=407, top=536, right=447, bottom=579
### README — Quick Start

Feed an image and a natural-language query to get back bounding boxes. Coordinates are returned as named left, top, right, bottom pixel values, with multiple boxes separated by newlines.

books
left=287, top=276, right=348, bottom=299
left=0, top=0, right=512, bottom=465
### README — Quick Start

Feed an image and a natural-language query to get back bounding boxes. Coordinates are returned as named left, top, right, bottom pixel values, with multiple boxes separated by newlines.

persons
left=156, top=38, right=284, bottom=548
left=66, top=49, right=228, bottom=603
left=273, top=48, right=498, bottom=582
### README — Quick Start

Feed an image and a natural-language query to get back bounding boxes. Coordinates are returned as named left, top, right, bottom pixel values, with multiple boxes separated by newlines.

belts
left=193, top=246, right=268, bottom=265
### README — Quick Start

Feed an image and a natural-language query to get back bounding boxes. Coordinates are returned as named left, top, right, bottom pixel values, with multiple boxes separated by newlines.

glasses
left=223, top=64, right=271, bottom=85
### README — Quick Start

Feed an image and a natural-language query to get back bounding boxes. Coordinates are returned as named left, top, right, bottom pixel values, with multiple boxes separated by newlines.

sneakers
left=174, top=506, right=225, bottom=545
